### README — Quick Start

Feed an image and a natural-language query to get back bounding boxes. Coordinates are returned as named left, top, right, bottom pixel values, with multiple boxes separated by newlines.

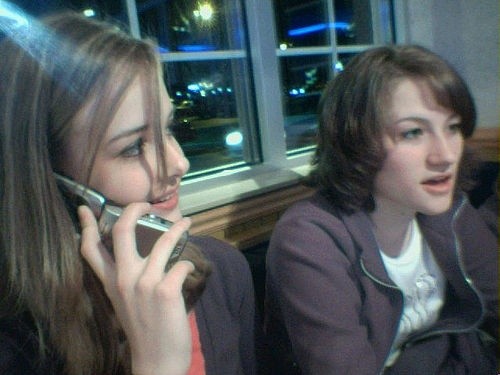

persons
left=263, top=46, right=500, bottom=375
left=0, top=12, right=258, bottom=375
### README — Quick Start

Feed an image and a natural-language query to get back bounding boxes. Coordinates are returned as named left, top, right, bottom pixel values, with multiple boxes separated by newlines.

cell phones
left=51, top=171, right=188, bottom=268
